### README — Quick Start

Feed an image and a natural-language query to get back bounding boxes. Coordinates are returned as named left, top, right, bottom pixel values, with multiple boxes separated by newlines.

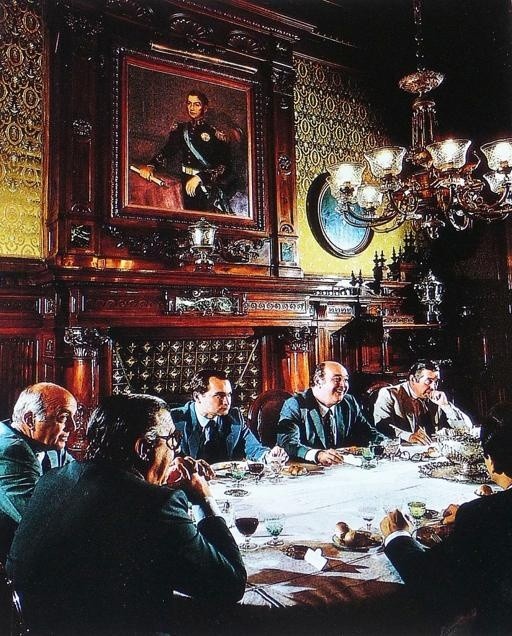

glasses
left=146, top=429, right=183, bottom=450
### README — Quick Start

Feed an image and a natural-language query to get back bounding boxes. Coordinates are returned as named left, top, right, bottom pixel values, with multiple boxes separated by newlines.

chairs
left=248, top=387, right=306, bottom=449
left=362, top=381, right=397, bottom=421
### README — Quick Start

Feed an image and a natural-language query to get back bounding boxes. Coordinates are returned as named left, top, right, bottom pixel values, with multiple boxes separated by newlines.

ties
left=324, top=409, right=336, bottom=449
left=206, top=420, right=218, bottom=462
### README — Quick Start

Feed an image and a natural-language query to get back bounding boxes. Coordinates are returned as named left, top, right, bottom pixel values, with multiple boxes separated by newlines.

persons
left=171, top=370, right=290, bottom=481
left=276, top=360, right=392, bottom=465
left=7, top=393, right=246, bottom=636
left=138, top=89, right=246, bottom=216
left=372, top=359, right=472, bottom=446
left=1, top=382, right=79, bottom=560
left=378, top=400, right=511, bottom=635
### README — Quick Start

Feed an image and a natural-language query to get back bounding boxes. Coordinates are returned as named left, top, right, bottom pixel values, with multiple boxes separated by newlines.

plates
left=410, top=527, right=437, bottom=550
left=335, top=447, right=365, bottom=458
left=332, top=530, right=383, bottom=551
left=210, top=461, right=251, bottom=478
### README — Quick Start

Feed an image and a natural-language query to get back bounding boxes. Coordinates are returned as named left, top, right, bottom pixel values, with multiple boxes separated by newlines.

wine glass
left=359, top=495, right=380, bottom=534
left=248, top=459, right=266, bottom=486
left=362, top=436, right=401, bottom=471
left=407, top=494, right=427, bottom=527
left=231, top=461, right=248, bottom=494
left=264, top=512, right=285, bottom=546
left=233, top=502, right=261, bottom=551
left=267, top=456, right=289, bottom=485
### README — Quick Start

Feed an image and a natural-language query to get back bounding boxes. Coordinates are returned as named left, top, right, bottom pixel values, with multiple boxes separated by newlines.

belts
left=182, top=165, right=201, bottom=179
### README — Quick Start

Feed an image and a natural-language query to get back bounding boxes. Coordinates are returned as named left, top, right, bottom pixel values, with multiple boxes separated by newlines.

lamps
left=326, top=1, right=512, bottom=240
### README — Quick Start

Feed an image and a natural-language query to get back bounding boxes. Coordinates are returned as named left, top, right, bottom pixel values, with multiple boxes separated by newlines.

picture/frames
left=106, top=37, right=270, bottom=241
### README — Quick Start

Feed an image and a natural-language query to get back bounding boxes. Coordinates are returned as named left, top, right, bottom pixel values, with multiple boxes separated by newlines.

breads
left=336, top=521, right=368, bottom=545
left=476, top=485, right=491, bottom=496
left=288, top=466, right=310, bottom=475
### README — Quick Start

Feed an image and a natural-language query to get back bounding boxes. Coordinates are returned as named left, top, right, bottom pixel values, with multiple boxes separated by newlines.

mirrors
left=314, top=178, right=375, bottom=259
